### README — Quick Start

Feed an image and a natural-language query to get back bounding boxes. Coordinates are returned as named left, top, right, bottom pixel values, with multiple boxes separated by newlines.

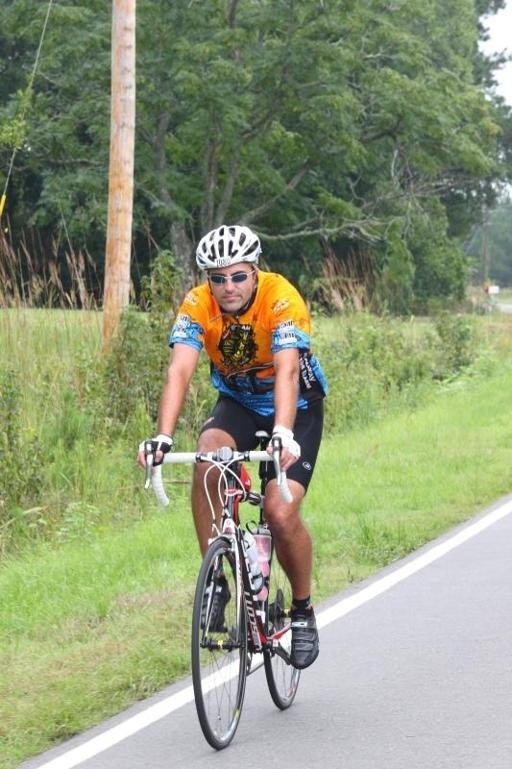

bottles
left=240, top=520, right=271, bottom=603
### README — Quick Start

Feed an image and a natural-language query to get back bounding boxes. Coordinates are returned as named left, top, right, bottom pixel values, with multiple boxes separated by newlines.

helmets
left=196, top=224, right=263, bottom=271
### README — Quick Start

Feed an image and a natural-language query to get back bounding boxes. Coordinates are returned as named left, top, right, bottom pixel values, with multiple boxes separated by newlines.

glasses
left=206, top=270, right=255, bottom=285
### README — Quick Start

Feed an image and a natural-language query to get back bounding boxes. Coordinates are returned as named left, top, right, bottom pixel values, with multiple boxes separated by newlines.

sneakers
left=200, top=576, right=231, bottom=631
left=288, top=604, right=320, bottom=669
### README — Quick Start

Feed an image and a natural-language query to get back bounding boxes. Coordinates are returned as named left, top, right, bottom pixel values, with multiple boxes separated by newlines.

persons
left=137, top=224, right=329, bottom=668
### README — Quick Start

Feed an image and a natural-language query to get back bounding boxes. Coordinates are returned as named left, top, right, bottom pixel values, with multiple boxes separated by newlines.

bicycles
left=142, top=429, right=301, bottom=750
